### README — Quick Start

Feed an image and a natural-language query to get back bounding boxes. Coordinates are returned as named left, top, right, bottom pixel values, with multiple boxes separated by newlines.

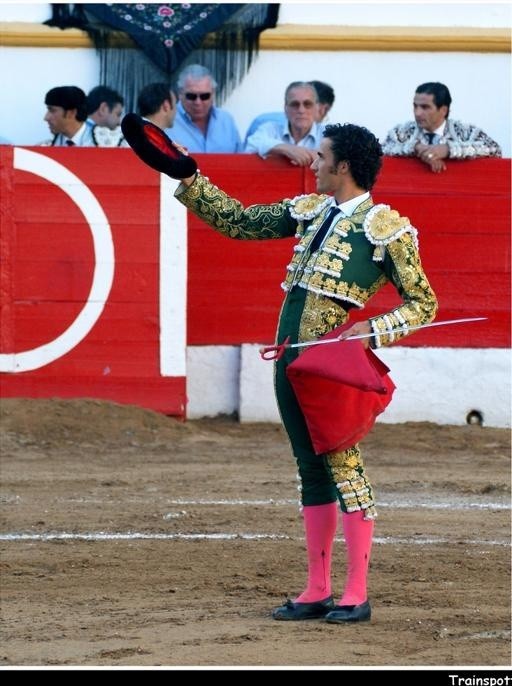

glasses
left=289, top=100, right=313, bottom=109
left=185, top=93, right=210, bottom=100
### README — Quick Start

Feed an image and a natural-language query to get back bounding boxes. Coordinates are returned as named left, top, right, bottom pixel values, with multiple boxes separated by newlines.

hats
left=121, top=112, right=197, bottom=179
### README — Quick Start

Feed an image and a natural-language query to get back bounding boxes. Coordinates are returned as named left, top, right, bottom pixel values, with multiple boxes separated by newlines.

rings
left=426, top=152, right=433, bottom=159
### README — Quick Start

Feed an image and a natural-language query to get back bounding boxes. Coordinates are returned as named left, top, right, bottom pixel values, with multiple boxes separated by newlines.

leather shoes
left=272, top=596, right=334, bottom=620
left=327, top=599, right=370, bottom=624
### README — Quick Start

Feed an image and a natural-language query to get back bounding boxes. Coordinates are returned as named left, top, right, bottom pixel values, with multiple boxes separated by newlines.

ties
left=426, top=133, right=436, bottom=144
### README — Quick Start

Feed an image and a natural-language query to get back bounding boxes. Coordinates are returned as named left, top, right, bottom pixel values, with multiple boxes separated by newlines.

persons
left=84, top=82, right=127, bottom=127
left=168, top=121, right=441, bottom=626
left=154, top=61, right=247, bottom=155
left=368, top=77, right=501, bottom=174
left=239, top=78, right=341, bottom=168
left=242, top=77, right=335, bottom=141
left=112, top=78, right=186, bottom=152
left=38, top=88, right=123, bottom=149
